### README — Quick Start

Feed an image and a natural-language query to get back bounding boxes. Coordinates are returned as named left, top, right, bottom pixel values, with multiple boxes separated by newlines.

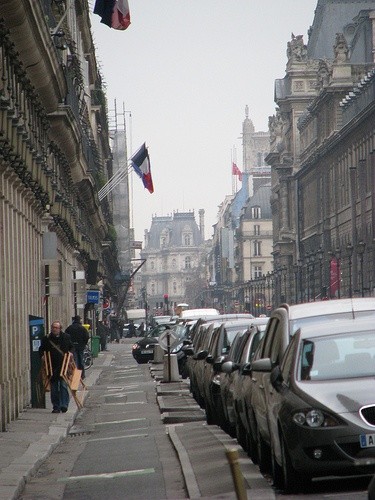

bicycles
left=83, top=351, right=93, bottom=370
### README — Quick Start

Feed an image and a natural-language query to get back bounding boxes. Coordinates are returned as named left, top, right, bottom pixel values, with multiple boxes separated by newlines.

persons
left=147, top=322, right=159, bottom=336
left=40, top=321, right=76, bottom=414
left=65, top=316, right=89, bottom=380
left=128, top=320, right=138, bottom=338
left=139, top=322, right=144, bottom=337
left=118, top=322, right=123, bottom=339
left=97, top=321, right=108, bottom=351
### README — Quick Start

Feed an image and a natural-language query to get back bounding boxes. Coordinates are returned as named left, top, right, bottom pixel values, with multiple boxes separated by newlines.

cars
left=248, top=317, right=375, bottom=493
left=123, top=298, right=375, bottom=462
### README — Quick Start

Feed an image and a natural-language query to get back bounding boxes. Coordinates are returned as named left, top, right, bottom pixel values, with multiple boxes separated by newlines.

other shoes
left=62, top=408, right=67, bottom=413
left=52, top=410, right=60, bottom=413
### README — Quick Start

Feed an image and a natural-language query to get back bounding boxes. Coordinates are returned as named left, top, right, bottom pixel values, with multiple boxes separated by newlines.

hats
left=75, top=316, right=82, bottom=320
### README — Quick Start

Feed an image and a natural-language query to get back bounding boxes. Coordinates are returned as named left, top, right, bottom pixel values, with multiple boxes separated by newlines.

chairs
left=60, top=352, right=87, bottom=409
left=35, top=351, right=53, bottom=403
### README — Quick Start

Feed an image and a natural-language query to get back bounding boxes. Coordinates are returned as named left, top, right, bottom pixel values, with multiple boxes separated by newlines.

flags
left=93, top=0, right=131, bottom=31
left=131, top=143, right=154, bottom=194
left=232, top=163, right=242, bottom=181
